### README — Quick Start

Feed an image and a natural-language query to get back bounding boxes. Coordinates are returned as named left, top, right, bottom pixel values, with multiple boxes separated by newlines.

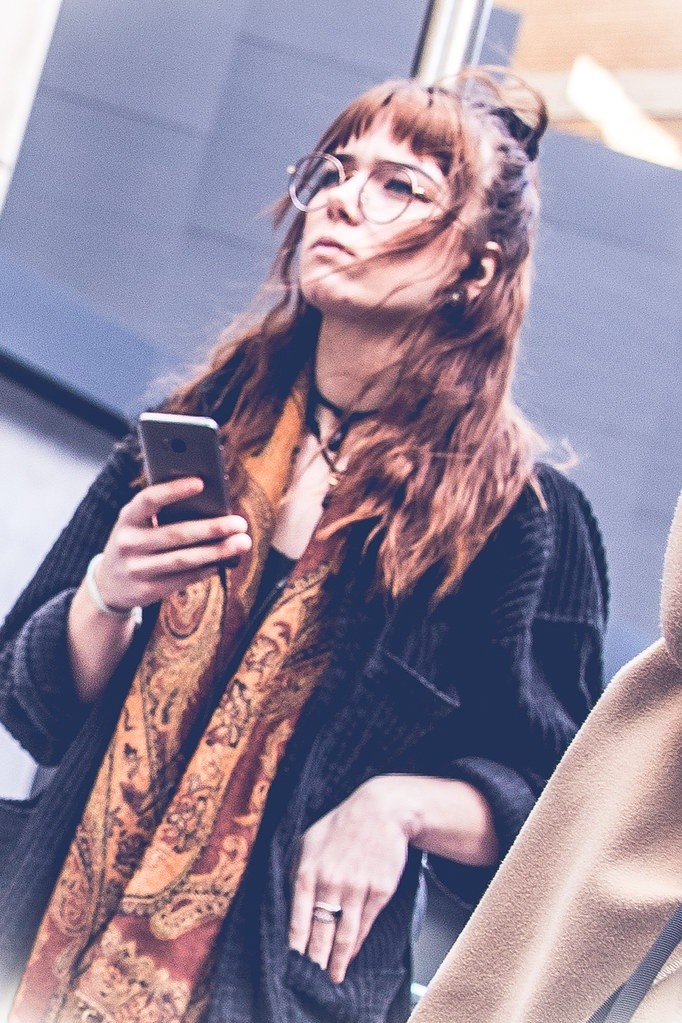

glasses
left=284, top=151, right=469, bottom=232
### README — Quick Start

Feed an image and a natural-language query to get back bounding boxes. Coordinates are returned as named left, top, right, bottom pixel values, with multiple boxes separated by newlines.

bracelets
left=85, top=553, right=132, bottom=619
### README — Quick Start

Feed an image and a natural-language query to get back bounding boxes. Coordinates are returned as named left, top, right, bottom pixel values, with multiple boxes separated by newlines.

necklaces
left=309, top=384, right=380, bottom=451
left=306, top=413, right=344, bottom=509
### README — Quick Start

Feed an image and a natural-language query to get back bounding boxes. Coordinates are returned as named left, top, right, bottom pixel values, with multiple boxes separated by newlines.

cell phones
left=137, top=413, right=239, bottom=567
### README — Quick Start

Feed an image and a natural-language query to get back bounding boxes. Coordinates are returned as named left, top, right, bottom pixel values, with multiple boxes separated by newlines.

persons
left=1, top=63, right=608, bottom=1023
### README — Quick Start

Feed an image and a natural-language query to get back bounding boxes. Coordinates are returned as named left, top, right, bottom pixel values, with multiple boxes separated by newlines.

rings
left=313, top=910, right=335, bottom=922
left=313, top=900, right=342, bottom=913
